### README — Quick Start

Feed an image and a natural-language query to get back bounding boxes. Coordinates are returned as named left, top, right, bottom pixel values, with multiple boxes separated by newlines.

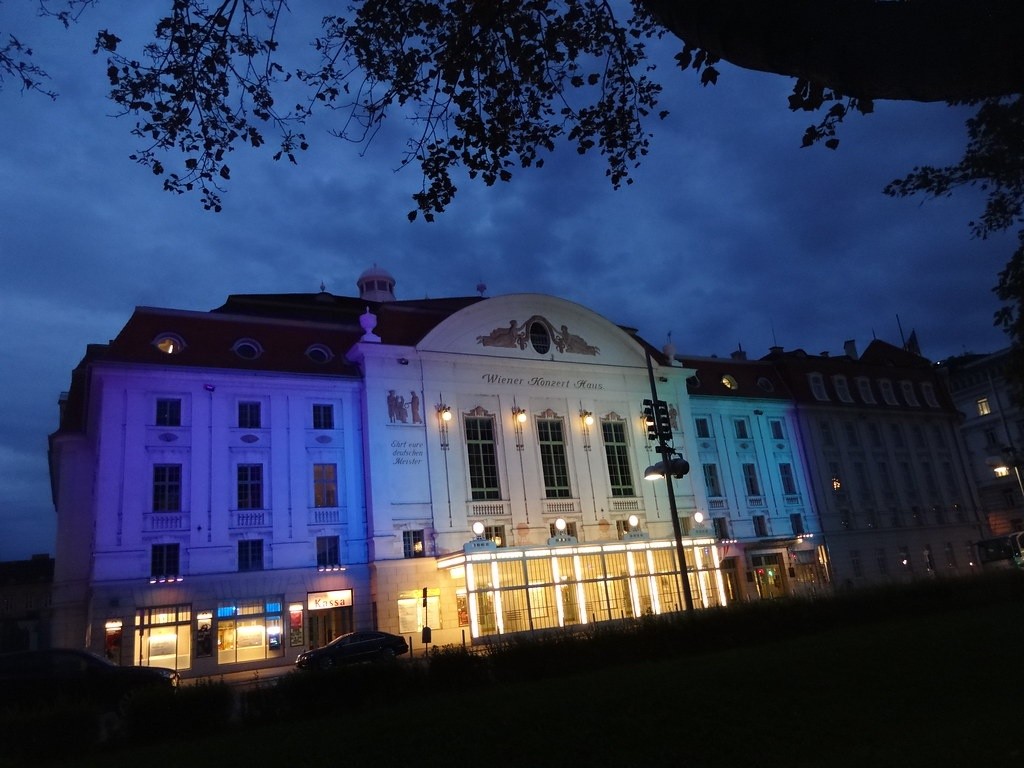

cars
left=0, top=645, right=183, bottom=721
left=293, top=630, right=409, bottom=674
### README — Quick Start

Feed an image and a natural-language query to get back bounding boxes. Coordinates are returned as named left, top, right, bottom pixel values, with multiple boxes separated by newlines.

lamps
left=628, top=515, right=640, bottom=533
left=150, top=575, right=183, bottom=584
left=318, top=565, right=347, bottom=572
left=580, top=410, right=593, bottom=426
left=437, top=404, right=453, bottom=421
left=472, top=521, right=485, bottom=540
left=512, top=407, right=527, bottom=423
left=693, top=511, right=704, bottom=529
left=554, top=518, right=568, bottom=536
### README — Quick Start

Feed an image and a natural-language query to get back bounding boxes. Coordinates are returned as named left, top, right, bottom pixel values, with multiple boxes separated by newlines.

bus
left=969, top=530, right=1024, bottom=579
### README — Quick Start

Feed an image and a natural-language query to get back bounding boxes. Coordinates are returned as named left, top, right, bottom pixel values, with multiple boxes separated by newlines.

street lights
left=982, top=440, right=1023, bottom=499
